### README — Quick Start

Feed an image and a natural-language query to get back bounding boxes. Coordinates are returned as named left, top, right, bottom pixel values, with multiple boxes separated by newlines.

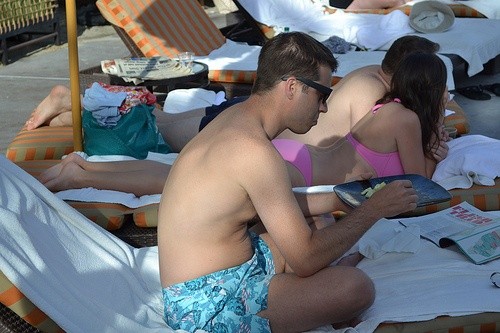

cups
left=178, top=51, right=194, bottom=71
left=444, top=127, right=457, bottom=138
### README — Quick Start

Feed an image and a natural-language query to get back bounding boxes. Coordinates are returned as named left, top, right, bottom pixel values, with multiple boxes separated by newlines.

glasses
left=490, top=272, right=500, bottom=288
left=281, top=76, right=333, bottom=104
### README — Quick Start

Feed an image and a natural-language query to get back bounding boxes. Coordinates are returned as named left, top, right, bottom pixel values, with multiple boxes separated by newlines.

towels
left=0, top=153, right=500, bottom=333
left=237, top=0, right=500, bottom=78
left=195, top=37, right=456, bottom=92
left=163, top=87, right=227, bottom=114
left=51, top=133, right=500, bottom=209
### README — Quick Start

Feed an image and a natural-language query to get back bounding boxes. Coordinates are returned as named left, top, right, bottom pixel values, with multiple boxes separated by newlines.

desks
left=110, top=61, right=210, bottom=101
left=206, top=12, right=245, bottom=35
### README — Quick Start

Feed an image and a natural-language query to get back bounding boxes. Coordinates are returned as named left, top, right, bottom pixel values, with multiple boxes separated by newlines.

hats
left=408, top=1, right=455, bottom=34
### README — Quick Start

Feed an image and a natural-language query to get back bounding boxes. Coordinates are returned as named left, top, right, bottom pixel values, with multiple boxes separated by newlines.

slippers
left=456, top=84, right=491, bottom=100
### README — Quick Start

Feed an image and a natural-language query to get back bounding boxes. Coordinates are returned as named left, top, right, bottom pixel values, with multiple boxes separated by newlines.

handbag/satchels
left=81, top=103, right=172, bottom=160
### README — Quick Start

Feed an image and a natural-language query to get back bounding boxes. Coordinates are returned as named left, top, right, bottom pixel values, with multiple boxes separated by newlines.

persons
left=158, top=32, right=418, bottom=333
left=329, top=0, right=411, bottom=12
left=26, top=35, right=440, bottom=147
left=37, top=51, right=450, bottom=198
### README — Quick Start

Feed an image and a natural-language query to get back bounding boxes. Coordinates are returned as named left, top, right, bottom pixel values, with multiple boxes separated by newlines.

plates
left=333, top=174, right=453, bottom=210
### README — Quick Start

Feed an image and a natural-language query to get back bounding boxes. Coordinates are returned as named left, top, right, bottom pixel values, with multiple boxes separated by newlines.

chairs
left=1, top=0, right=500, bottom=333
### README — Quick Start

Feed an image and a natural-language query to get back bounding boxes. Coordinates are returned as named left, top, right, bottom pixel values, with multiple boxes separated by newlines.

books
left=399, top=200, right=500, bottom=265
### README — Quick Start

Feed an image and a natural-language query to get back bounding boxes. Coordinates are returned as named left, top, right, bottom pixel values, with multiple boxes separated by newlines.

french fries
left=361, top=182, right=387, bottom=197
